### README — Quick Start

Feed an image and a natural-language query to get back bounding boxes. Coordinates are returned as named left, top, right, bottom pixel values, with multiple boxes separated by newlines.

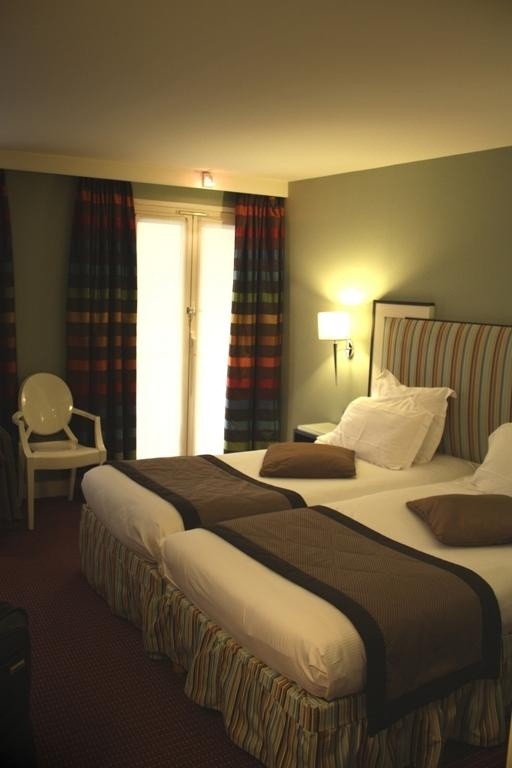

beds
left=155, top=474, right=512, bottom=767
left=79, top=316, right=512, bottom=653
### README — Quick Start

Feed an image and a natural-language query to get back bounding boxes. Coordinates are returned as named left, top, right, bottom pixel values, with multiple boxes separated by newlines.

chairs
left=12, top=370, right=107, bottom=531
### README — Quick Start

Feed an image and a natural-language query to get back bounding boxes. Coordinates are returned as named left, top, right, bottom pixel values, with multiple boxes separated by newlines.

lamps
left=201, top=170, right=215, bottom=187
left=317, top=312, right=354, bottom=360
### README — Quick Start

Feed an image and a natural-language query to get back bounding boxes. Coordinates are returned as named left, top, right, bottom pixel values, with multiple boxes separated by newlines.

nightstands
left=292, top=422, right=337, bottom=442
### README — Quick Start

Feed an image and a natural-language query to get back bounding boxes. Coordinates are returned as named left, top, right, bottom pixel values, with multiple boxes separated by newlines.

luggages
left=0, top=600, right=31, bottom=742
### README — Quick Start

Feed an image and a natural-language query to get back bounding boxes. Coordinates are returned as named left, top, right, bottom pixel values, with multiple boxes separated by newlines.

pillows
left=376, top=367, right=458, bottom=464
left=332, top=396, right=433, bottom=472
left=259, top=442, right=357, bottom=478
left=404, top=493, right=511, bottom=548
left=471, top=421, right=512, bottom=493
